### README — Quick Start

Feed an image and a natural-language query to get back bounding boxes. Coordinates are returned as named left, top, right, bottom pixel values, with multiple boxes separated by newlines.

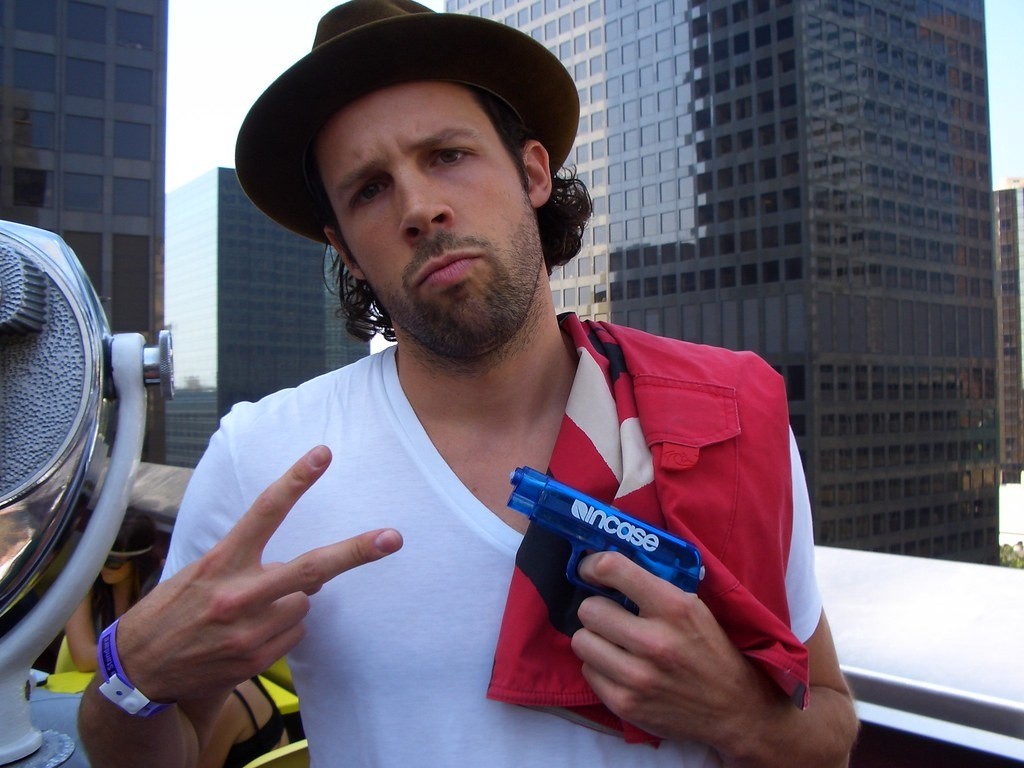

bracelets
left=98, top=618, right=172, bottom=718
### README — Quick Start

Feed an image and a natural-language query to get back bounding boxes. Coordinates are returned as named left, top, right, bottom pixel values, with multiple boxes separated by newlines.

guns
left=505, top=464, right=706, bottom=615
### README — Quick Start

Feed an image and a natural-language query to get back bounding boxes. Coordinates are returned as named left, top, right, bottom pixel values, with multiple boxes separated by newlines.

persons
left=77, top=0, right=859, bottom=768
left=55, top=506, right=166, bottom=675
left=201, top=676, right=290, bottom=768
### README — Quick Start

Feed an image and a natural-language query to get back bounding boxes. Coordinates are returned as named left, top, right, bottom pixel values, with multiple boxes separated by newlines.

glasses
left=105, top=555, right=132, bottom=569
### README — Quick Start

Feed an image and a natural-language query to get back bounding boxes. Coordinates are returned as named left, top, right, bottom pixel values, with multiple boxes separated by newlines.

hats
left=234, top=0, right=582, bottom=243
